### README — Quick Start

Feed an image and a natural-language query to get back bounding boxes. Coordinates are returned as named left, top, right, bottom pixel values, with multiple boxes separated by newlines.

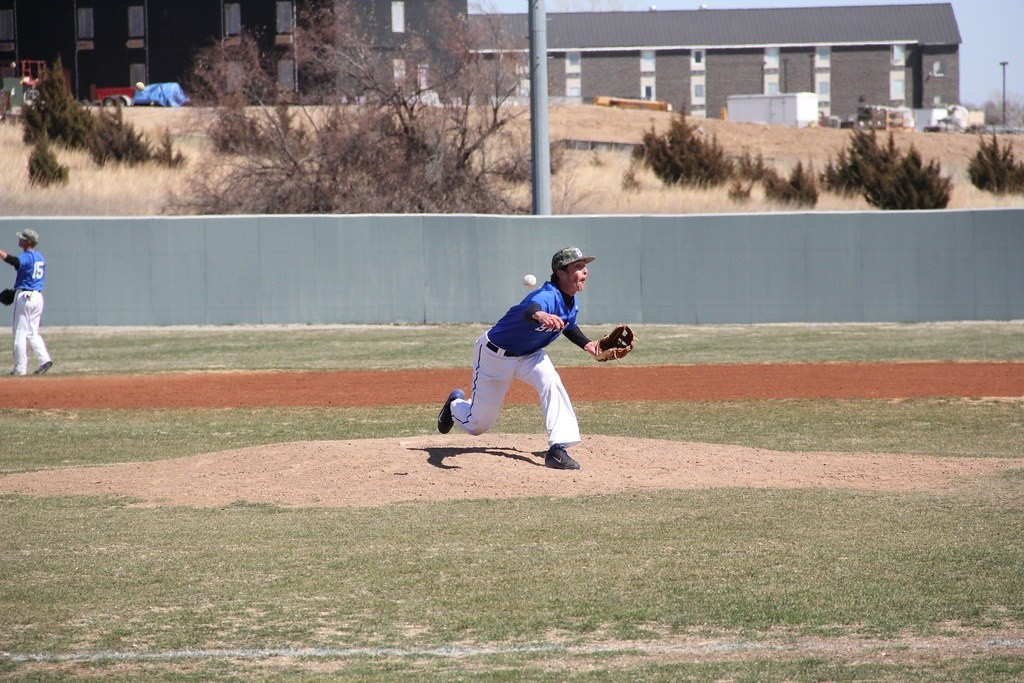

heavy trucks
left=90, top=83, right=189, bottom=112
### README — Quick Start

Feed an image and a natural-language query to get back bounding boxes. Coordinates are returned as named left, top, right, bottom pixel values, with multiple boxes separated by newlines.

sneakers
left=544, top=448, right=580, bottom=469
left=438, top=389, right=464, bottom=434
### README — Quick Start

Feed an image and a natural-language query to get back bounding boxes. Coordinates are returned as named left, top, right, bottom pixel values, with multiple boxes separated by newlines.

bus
left=589, top=94, right=674, bottom=111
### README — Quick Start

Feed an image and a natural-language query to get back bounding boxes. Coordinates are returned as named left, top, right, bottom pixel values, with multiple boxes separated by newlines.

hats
left=552, top=247, right=595, bottom=274
left=16, top=229, right=38, bottom=244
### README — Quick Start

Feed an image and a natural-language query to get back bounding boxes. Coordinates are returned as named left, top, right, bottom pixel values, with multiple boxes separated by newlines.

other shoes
left=34, top=361, right=52, bottom=376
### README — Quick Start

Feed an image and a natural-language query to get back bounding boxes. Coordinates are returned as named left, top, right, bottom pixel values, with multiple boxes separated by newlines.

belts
left=23, top=289, right=41, bottom=292
left=487, top=342, right=520, bottom=356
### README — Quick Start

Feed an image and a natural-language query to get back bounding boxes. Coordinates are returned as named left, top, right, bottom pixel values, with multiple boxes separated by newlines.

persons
left=0, top=229, right=53, bottom=376
left=438, top=246, right=634, bottom=470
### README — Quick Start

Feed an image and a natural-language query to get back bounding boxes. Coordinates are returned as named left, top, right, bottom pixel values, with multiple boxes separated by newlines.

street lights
left=1000, top=61, right=1010, bottom=122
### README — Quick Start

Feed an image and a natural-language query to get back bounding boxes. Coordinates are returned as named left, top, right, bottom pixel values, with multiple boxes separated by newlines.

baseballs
left=523, top=274, right=537, bottom=287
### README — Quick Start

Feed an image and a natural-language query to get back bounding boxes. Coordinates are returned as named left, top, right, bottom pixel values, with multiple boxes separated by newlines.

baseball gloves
left=594, top=322, right=640, bottom=366
left=0, top=289, right=15, bottom=306
